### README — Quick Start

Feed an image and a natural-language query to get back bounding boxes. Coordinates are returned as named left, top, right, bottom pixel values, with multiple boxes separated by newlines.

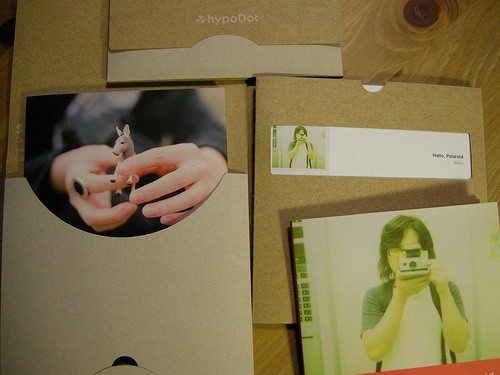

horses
left=110, top=123, right=138, bottom=199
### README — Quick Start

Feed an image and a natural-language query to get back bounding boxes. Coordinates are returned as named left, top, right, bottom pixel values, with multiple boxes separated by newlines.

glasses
left=387, top=247, right=402, bottom=257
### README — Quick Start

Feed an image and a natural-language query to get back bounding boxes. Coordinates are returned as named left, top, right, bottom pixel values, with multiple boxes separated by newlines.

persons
left=359, top=214, right=470, bottom=374
left=285, top=126, right=317, bottom=168
left=25, top=89, right=228, bottom=236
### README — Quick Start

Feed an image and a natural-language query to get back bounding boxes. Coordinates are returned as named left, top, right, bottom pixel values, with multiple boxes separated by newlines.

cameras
left=297, top=133, right=307, bottom=140
left=398, top=245, right=429, bottom=276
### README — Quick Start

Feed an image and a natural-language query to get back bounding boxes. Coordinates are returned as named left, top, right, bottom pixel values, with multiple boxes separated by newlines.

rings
left=71, top=175, right=89, bottom=199
